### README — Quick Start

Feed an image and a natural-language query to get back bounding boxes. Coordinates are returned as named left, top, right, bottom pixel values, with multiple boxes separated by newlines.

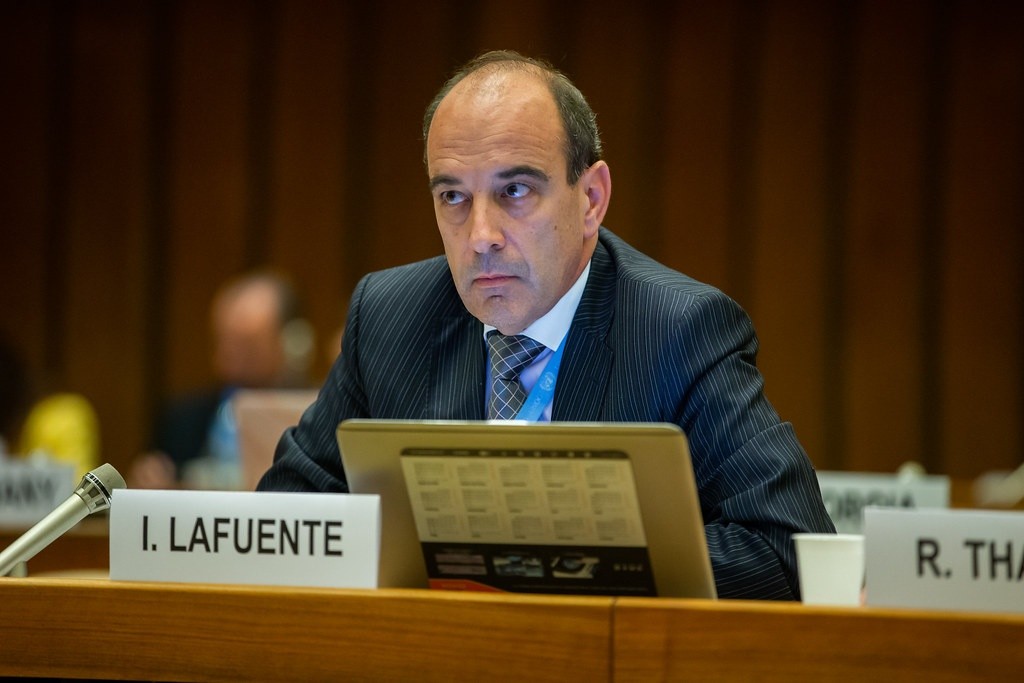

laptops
left=337, top=417, right=718, bottom=603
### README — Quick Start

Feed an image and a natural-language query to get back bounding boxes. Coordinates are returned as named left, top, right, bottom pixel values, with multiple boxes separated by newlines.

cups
left=791, top=533, right=867, bottom=606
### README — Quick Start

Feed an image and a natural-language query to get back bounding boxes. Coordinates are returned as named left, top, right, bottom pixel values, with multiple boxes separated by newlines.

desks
left=0, top=476, right=1024, bottom=683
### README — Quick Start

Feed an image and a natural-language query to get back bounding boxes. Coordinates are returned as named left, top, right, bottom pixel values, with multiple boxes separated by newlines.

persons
left=253, top=47, right=838, bottom=603
left=127, top=254, right=309, bottom=494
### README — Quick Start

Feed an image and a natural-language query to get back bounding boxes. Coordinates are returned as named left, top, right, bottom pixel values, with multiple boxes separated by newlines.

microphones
left=0, top=463, right=128, bottom=576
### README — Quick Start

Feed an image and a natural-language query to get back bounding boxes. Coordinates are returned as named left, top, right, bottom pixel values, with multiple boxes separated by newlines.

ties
left=487, top=330, right=547, bottom=420
